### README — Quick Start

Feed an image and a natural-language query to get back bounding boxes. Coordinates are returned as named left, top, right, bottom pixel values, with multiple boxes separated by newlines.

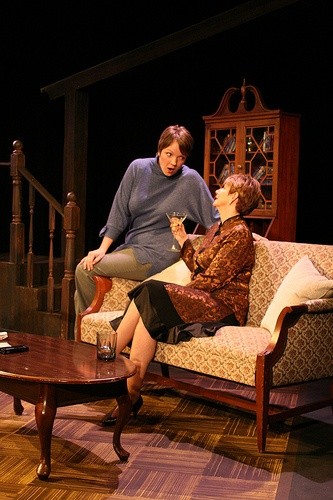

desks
left=0, top=332, right=138, bottom=479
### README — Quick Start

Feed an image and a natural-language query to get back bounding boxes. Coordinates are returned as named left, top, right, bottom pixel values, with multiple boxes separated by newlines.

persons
left=73, top=125, right=268, bottom=341
left=101, top=174, right=262, bottom=425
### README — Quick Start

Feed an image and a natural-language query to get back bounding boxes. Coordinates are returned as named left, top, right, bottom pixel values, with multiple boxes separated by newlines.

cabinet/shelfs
left=204, top=79, right=301, bottom=242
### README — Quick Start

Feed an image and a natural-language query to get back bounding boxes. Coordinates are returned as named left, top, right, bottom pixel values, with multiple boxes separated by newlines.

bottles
left=247, top=136, right=252, bottom=152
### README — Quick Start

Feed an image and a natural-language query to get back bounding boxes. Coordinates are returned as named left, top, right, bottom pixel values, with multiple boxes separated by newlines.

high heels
left=102, top=395, right=143, bottom=426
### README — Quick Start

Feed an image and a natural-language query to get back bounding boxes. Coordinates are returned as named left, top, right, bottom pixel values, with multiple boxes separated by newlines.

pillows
left=141, top=259, right=192, bottom=286
left=260, top=254, right=333, bottom=335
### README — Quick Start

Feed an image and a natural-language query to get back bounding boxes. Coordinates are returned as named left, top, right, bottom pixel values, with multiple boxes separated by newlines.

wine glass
left=165, top=208, right=187, bottom=252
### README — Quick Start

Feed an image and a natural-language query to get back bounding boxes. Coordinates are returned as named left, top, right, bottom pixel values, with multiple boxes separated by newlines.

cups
left=262, top=132, right=271, bottom=151
left=96, top=331, right=117, bottom=361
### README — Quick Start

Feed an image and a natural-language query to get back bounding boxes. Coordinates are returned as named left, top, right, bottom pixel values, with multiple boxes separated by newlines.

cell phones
left=0, top=345, right=29, bottom=354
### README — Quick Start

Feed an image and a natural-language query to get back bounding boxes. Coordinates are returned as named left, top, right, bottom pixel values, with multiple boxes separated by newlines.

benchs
left=76, top=241, right=333, bottom=453
